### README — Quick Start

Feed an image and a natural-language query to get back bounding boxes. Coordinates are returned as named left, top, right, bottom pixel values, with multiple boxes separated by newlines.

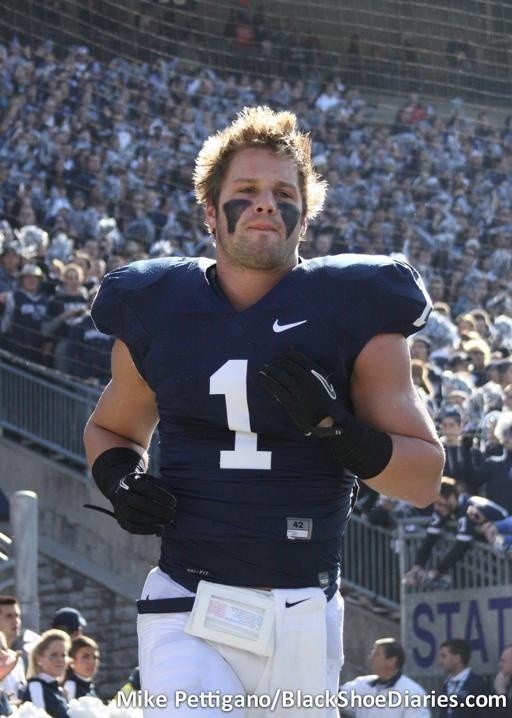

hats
left=53, top=606, right=87, bottom=631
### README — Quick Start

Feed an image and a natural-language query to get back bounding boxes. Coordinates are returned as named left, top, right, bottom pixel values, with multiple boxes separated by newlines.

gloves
left=91, top=447, right=177, bottom=536
left=260, top=350, right=392, bottom=478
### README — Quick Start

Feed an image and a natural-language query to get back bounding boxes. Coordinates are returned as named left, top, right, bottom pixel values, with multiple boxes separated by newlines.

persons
left=5, top=2, right=511, bottom=716
